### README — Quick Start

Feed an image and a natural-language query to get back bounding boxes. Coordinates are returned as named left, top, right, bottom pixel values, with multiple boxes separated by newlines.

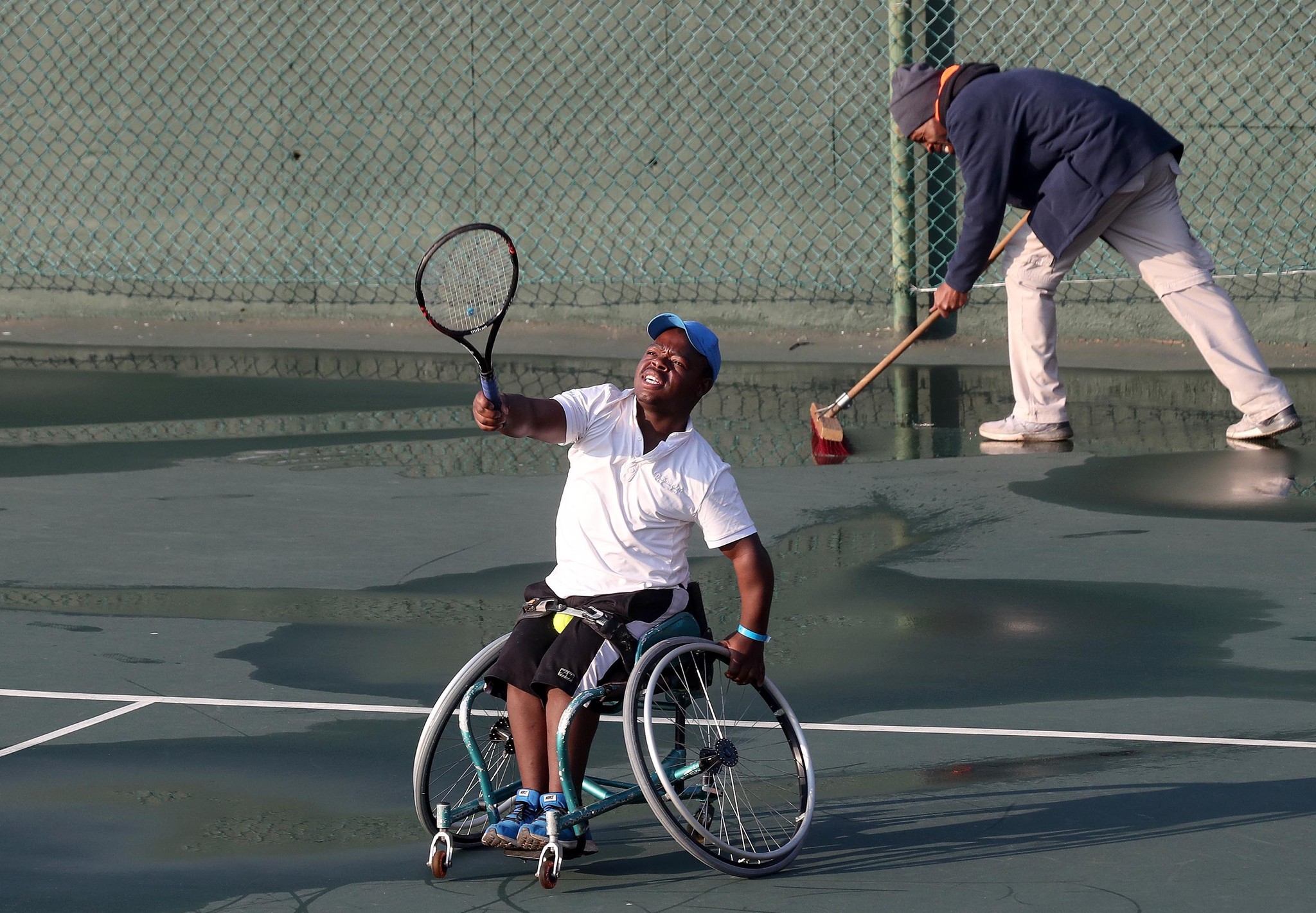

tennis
left=552, top=612, right=574, bottom=635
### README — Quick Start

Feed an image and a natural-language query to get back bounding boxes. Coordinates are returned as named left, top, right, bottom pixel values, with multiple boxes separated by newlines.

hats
left=889, top=61, right=943, bottom=139
left=647, top=312, right=722, bottom=383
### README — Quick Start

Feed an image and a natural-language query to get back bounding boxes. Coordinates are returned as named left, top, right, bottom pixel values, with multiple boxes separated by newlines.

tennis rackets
left=413, top=225, right=518, bottom=416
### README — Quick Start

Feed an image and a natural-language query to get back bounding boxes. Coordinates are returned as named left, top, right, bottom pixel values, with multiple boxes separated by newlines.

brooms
left=804, top=211, right=1028, bottom=463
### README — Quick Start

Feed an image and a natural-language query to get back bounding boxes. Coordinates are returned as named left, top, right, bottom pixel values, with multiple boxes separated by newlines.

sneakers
left=481, top=788, right=542, bottom=848
left=1233, top=477, right=1293, bottom=498
left=1227, top=440, right=1281, bottom=450
left=517, top=791, right=600, bottom=856
left=979, top=415, right=1074, bottom=440
left=1226, top=403, right=1302, bottom=439
left=980, top=442, right=1074, bottom=455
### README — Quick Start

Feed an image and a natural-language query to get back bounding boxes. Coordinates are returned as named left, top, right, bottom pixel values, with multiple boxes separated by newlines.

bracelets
left=737, top=624, right=771, bottom=643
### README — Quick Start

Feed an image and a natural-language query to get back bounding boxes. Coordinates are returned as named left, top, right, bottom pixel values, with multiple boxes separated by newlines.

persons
left=889, top=63, right=1301, bottom=443
left=472, top=312, right=774, bottom=851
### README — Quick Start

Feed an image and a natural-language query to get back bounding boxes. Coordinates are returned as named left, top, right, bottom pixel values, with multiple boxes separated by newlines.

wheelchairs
left=413, top=583, right=823, bottom=889
